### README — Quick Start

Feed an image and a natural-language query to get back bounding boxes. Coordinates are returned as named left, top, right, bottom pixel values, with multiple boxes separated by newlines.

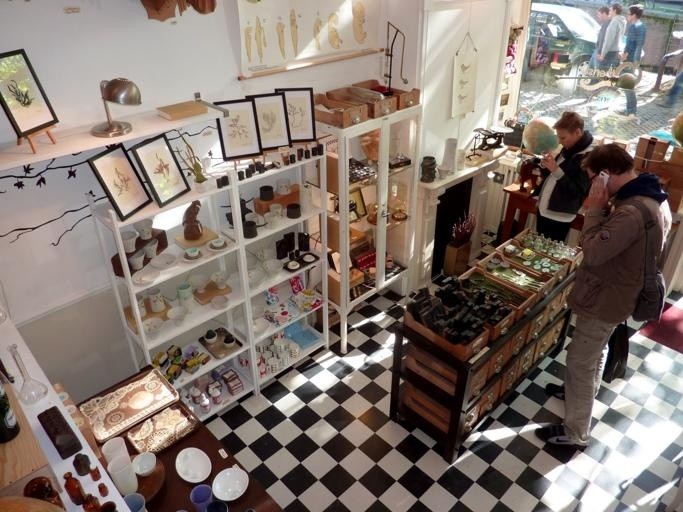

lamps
left=88, top=77, right=141, bottom=138
left=502, top=70, right=640, bottom=130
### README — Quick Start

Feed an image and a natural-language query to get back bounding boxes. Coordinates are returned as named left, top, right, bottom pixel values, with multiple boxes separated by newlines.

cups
left=207, top=502, right=226, bottom=511
left=189, top=484, right=211, bottom=510
left=114, top=139, right=322, bottom=373
left=420, top=137, right=465, bottom=182
left=124, top=494, right=148, bottom=512
left=106, top=455, right=138, bottom=495
left=100, top=436, right=126, bottom=462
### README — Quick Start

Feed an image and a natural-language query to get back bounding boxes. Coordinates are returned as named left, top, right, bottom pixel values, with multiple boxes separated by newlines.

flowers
left=448, top=211, right=477, bottom=246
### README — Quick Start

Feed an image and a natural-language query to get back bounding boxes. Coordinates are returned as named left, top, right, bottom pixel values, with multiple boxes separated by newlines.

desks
left=500, top=180, right=585, bottom=246
left=66, top=368, right=284, bottom=512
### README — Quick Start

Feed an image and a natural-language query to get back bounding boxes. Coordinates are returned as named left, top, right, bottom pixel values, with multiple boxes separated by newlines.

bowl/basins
left=131, top=452, right=155, bottom=477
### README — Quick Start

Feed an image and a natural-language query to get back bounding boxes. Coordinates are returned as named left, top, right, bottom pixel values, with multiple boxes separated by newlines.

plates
left=174, top=448, right=210, bottom=484
left=212, top=466, right=254, bottom=499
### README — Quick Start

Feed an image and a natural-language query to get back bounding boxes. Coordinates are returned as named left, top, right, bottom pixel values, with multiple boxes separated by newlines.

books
left=156, top=100, right=208, bottom=122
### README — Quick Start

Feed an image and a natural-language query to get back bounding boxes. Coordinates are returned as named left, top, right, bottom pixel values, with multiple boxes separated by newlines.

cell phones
left=599, top=171, right=610, bottom=188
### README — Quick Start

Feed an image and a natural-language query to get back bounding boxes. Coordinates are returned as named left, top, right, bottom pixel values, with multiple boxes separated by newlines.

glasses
left=584, top=173, right=605, bottom=182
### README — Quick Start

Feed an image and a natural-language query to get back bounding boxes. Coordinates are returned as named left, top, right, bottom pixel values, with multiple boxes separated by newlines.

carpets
left=639, top=300, right=683, bottom=356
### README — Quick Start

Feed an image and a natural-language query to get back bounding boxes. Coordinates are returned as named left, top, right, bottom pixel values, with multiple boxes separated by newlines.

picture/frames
left=213, top=100, right=261, bottom=164
left=345, top=187, right=368, bottom=217
left=0, top=46, right=59, bottom=153
left=245, top=91, right=291, bottom=159
left=132, top=130, right=191, bottom=208
left=87, top=142, right=150, bottom=224
left=274, top=87, right=316, bottom=146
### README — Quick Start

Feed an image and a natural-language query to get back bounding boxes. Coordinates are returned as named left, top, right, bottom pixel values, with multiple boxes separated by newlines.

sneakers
left=533, top=423, right=590, bottom=453
left=545, top=378, right=565, bottom=403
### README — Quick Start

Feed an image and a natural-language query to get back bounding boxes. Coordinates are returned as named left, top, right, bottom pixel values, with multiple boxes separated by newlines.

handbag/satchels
left=633, top=268, right=667, bottom=326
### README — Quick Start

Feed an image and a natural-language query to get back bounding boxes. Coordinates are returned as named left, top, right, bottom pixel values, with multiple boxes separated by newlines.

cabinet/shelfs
left=390, top=228, right=584, bottom=457
left=315, top=103, right=423, bottom=352
left=0, top=305, right=129, bottom=512
left=85, top=158, right=258, bottom=423
left=207, top=138, right=327, bottom=395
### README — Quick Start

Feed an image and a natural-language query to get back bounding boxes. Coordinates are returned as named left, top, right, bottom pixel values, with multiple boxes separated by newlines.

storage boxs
left=628, top=136, right=683, bottom=213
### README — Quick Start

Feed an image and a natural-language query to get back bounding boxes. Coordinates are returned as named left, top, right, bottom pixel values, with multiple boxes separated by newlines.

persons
left=536, top=110, right=600, bottom=242
left=619, top=4, right=647, bottom=116
left=589, top=2, right=627, bottom=84
left=671, top=113, right=683, bottom=148
left=657, top=46, right=683, bottom=109
left=535, top=142, right=676, bottom=449
left=588, top=6, right=612, bottom=70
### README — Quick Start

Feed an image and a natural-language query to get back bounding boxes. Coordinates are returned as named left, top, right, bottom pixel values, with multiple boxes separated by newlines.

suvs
left=526, top=2, right=645, bottom=76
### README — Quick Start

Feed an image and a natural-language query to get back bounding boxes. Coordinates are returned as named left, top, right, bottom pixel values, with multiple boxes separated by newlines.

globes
left=522, top=116, right=558, bottom=158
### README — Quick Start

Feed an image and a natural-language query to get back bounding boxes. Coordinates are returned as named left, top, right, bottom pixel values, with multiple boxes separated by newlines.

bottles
left=8, top=344, right=47, bottom=404
left=22, top=476, right=58, bottom=509
left=180, top=386, right=223, bottom=415
left=60, top=453, right=117, bottom=511
left=482, top=227, right=573, bottom=290
left=0, top=383, right=20, bottom=445
